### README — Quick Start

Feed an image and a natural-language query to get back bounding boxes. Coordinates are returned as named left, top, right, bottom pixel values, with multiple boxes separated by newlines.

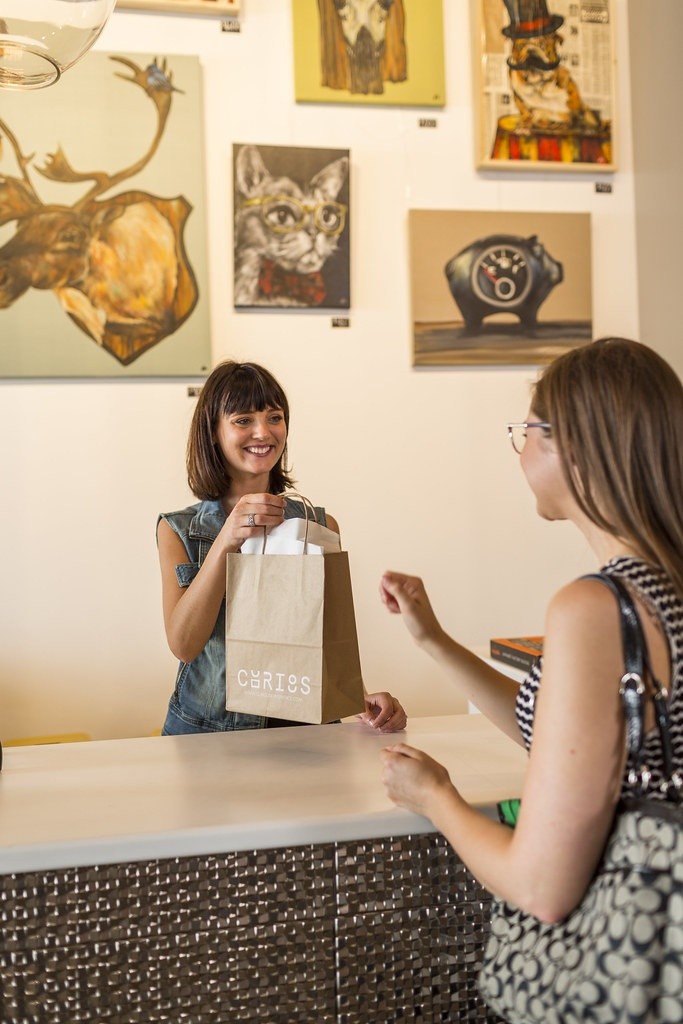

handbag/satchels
left=225, top=493, right=366, bottom=724
left=473, top=574, right=683, bottom=1024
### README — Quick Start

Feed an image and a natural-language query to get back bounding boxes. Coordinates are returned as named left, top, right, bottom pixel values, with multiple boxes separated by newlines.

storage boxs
left=489, top=635, right=543, bottom=671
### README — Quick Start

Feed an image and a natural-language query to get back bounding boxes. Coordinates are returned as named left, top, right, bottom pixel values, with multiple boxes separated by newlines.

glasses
left=507, top=422, right=551, bottom=454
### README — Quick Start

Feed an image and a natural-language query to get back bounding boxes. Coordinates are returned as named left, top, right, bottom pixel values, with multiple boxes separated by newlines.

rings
left=248, top=514, right=255, bottom=525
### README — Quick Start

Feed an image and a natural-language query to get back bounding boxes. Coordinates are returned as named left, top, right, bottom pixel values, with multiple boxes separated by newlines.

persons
left=156, top=362, right=408, bottom=735
left=378, top=338, right=683, bottom=925
